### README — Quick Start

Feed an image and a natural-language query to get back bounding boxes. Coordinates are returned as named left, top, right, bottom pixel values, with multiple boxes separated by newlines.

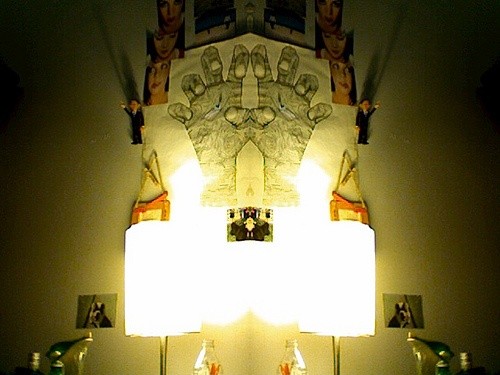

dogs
left=387, top=302, right=412, bottom=328
left=91, top=300, right=113, bottom=327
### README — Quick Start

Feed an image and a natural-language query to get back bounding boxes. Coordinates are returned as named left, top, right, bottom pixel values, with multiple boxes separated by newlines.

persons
left=157, top=0, right=184, bottom=30
left=314, top=0, right=342, bottom=34
left=320, top=30, right=349, bottom=64
left=154, top=30, right=181, bottom=61
left=330, top=63, right=358, bottom=106
left=355, top=99, right=379, bottom=144
left=121, top=100, right=145, bottom=145
left=145, top=60, right=170, bottom=106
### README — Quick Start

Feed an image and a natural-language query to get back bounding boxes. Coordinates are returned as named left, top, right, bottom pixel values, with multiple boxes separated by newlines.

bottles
left=278, top=341, right=306, bottom=374
left=194, top=339, right=222, bottom=375
left=459, top=351, right=471, bottom=375
left=28, top=352, right=40, bottom=375
